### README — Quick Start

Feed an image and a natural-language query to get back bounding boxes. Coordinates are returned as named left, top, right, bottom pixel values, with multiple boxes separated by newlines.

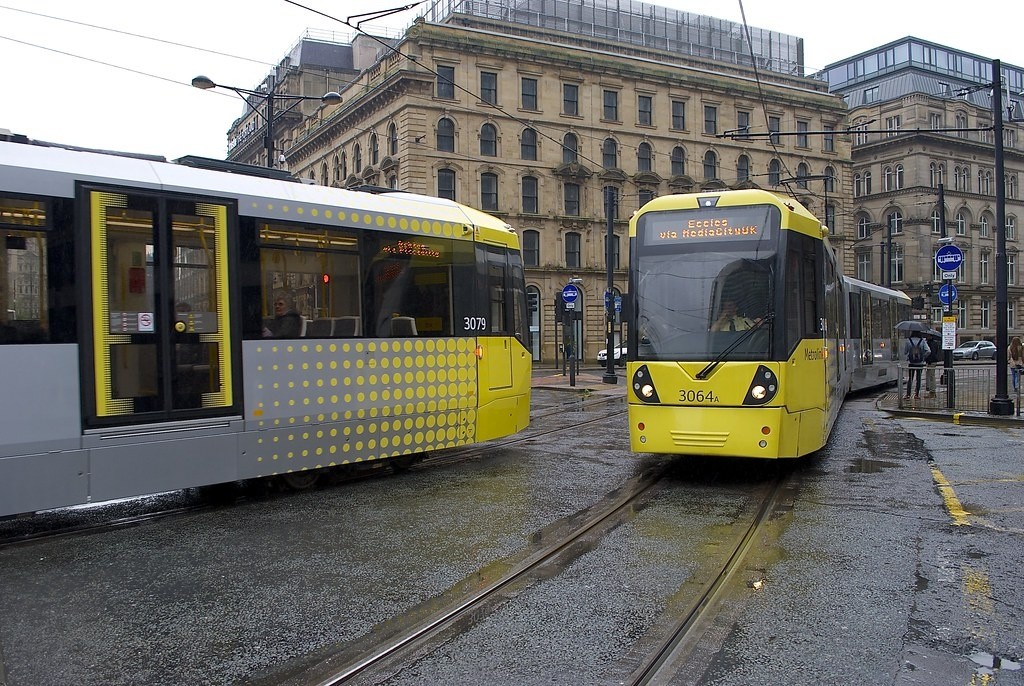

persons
left=262, top=290, right=302, bottom=338
left=1007, top=337, right=1023, bottom=392
left=904, top=331, right=931, bottom=400
left=923, top=334, right=938, bottom=398
left=707, top=299, right=763, bottom=333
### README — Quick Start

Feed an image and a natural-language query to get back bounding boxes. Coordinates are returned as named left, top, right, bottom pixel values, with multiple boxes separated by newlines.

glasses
left=274, top=303, right=284, bottom=306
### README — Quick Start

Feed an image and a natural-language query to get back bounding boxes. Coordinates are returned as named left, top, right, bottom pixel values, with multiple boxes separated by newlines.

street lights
left=191, top=76, right=343, bottom=167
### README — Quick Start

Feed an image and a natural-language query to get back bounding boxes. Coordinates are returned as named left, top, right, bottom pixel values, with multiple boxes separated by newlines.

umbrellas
left=894, top=321, right=930, bottom=337
left=921, top=328, right=941, bottom=338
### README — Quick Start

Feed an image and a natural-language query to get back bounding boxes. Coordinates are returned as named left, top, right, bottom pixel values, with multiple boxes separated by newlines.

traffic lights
left=554, top=298, right=562, bottom=322
left=526, top=289, right=536, bottom=317
left=323, top=272, right=329, bottom=285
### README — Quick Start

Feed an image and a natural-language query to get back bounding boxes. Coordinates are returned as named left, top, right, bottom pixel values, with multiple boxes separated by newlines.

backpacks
left=908, top=337, right=923, bottom=363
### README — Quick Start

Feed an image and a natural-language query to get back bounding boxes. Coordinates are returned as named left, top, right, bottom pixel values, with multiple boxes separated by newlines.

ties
left=729, top=319, right=736, bottom=331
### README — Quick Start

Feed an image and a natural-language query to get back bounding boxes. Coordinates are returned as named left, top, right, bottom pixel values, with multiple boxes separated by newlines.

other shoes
left=904, top=395, right=910, bottom=401
left=914, top=395, right=921, bottom=401
left=924, top=392, right=936, bottom=398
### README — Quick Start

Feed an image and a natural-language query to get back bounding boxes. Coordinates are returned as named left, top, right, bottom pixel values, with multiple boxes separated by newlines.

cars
left=597, top=340, right=628, bottom=367
left=953, top=341, right=997, bottom=360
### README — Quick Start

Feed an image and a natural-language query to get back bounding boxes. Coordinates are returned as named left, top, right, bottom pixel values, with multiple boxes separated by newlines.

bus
left=618, top=175, right=913, bottom=459
left=1, top=130, right=531, bottom=520
